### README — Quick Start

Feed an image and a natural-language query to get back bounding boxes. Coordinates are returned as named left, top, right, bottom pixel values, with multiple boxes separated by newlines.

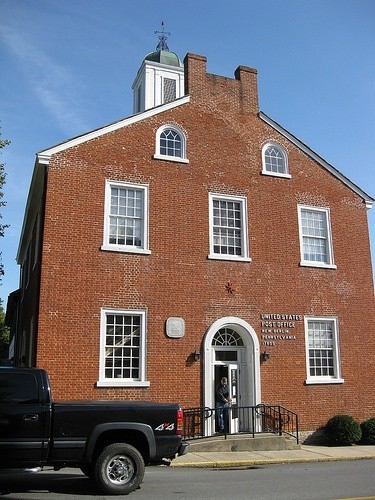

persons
left=215, top=377, right=232, bottom=435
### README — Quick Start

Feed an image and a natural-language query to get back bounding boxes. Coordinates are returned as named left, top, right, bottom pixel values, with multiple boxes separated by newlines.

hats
left=222, top=377, right=228, bottom=382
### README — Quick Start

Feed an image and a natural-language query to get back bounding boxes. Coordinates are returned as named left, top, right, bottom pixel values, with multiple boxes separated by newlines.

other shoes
left=218, top=429, right=224, bottom=436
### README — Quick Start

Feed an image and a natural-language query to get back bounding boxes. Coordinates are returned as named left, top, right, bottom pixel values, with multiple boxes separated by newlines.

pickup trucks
left=0, top=367, right=188, bottom=495
